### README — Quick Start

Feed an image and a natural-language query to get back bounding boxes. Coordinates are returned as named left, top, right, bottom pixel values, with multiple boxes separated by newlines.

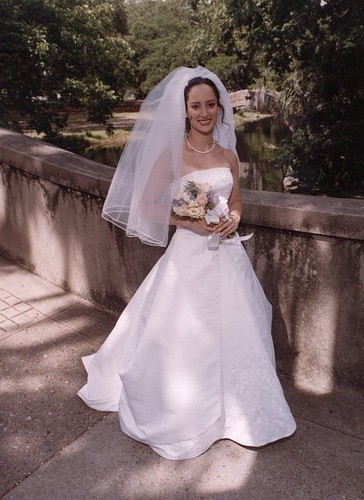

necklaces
left=187, top=139, right=216, bottom=154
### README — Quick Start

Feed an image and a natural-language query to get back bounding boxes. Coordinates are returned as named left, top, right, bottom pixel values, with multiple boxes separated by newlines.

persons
left=138, top=77, right=273, bottom=448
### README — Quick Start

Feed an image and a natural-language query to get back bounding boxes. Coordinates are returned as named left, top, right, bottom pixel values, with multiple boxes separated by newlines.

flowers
left=172, top=180, right=236, bottom=240
